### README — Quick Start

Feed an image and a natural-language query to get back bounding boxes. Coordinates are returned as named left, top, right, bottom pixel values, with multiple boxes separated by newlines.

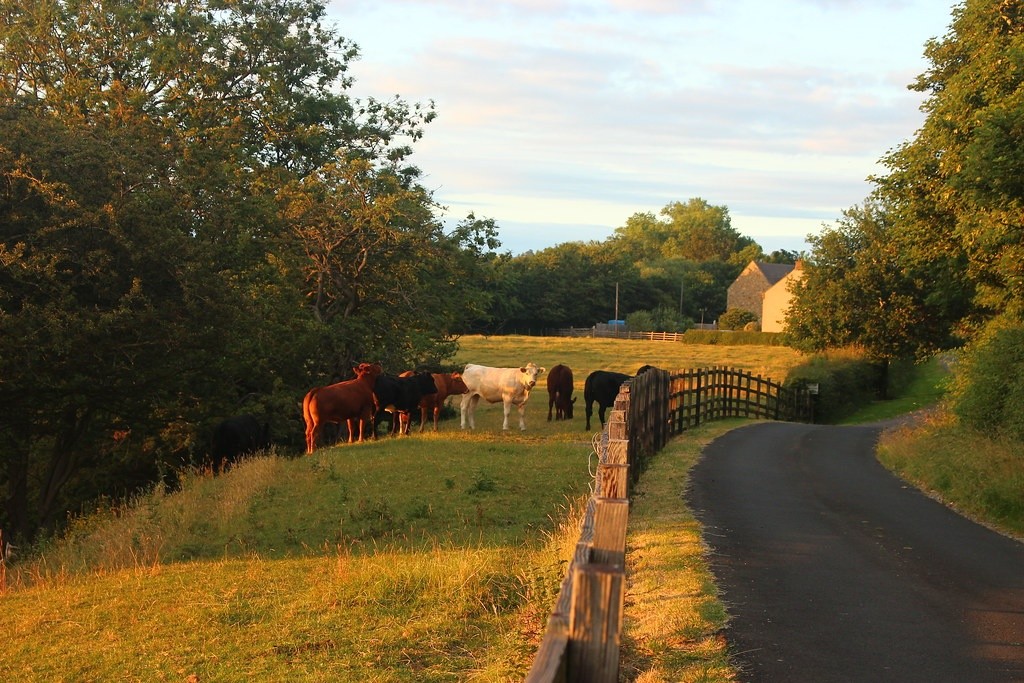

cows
left=371, top=372, right=438, bottom=441
left=460, top=363, right=546, bottom=430
left=302, top=360, right=383, bottom=454
left=398, top=371, right=469, bottom=435
left=584, top=370, right=636, bottom=431
left=547, top=364, right=577, bottom=422
